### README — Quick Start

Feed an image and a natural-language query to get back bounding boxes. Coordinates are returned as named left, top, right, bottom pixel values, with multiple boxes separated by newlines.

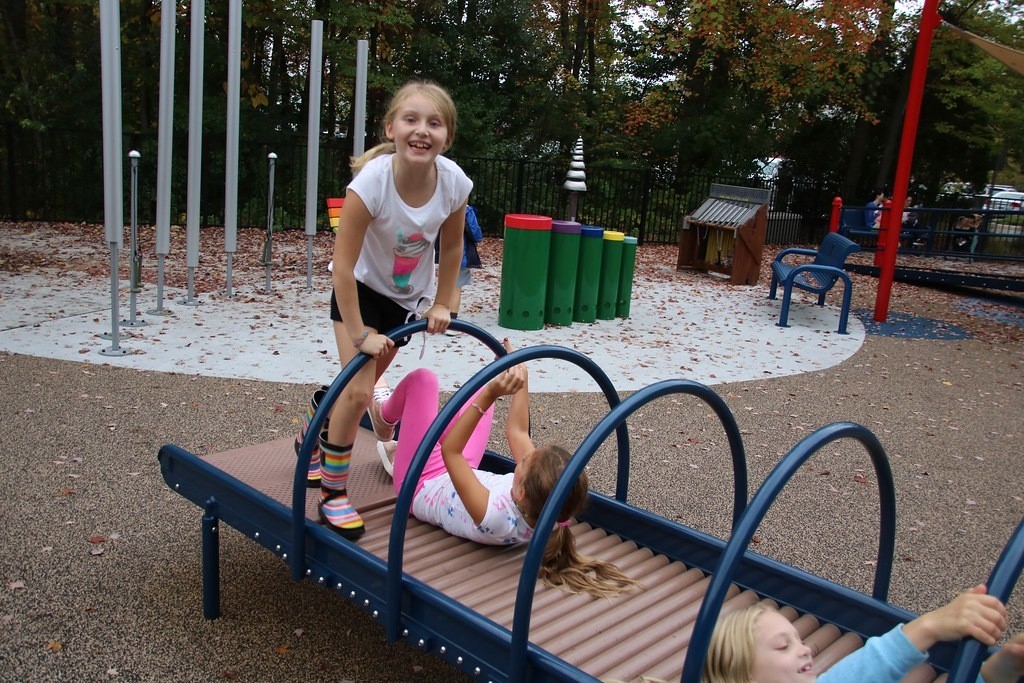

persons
left=294, top=82, right=469, bottom=539
left=606, top=583, right=1024, bottom=683
left=434, top=201, right=483, bottom=319
left=864, top=188, right=923, bottom=259
left=366, top=337, right=646, bottom=606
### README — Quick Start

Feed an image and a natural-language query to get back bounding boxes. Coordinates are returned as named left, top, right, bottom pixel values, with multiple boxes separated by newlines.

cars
left=981, top=191, right=1024, bottom=219
left=935, top=181, right=1018, bottom=210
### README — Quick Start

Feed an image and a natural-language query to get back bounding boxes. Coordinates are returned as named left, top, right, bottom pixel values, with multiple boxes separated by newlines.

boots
left=318, top=430, right=366, bottom=537
left=294, top=389, right=327, bottom=487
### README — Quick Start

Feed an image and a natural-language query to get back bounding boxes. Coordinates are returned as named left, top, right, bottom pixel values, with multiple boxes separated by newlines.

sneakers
left=366, top=375, right=398, bottom=442
left=377, top=439, right=398, bottom=479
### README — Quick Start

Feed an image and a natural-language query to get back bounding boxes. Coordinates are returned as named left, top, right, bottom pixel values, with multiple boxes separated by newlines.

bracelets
left=353, top=328, right=369, bottom=348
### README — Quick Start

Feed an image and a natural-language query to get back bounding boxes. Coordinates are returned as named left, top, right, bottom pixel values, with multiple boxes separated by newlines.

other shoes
left=444, top=328, right=462, bottom=336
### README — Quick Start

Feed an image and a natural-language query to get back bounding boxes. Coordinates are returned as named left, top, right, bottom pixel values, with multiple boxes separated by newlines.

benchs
left=767, top=232, right=862, bottom=333
left=325, top=197, right=344, bottom=272
left=839, top=207, right=931, bottom=240
